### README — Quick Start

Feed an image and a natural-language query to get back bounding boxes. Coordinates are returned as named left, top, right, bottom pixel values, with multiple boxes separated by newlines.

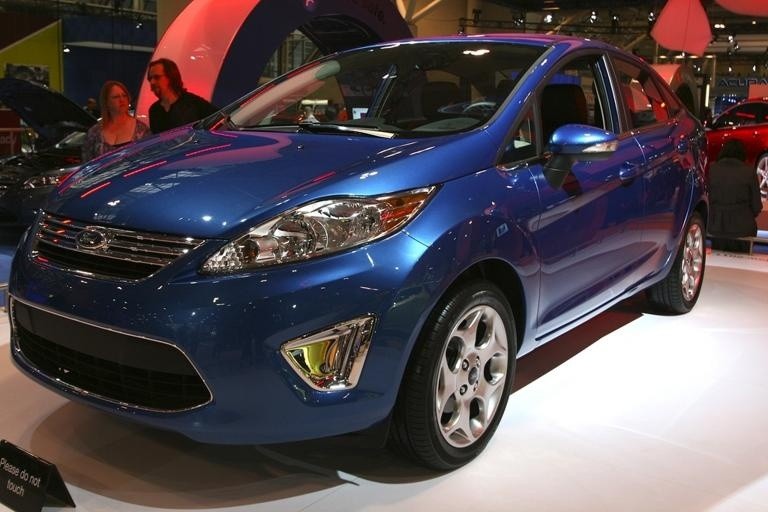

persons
left=83, top=98, right=96, bottom=115
left=82, top=80, right=151, bottom=159
left=708, top=138, right=762, bottom=251
left=147, top=56, right=217, bottom=135
left=300, top=107, right=320, bottom=124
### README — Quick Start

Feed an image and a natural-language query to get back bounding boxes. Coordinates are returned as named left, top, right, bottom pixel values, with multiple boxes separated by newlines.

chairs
left=391, top=81, right=463, bottom=120
left=499, top=81, right=587, bottom=159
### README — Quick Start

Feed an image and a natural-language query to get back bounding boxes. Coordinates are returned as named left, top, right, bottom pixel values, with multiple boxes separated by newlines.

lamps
left=472, top=7, right=758, bottom=33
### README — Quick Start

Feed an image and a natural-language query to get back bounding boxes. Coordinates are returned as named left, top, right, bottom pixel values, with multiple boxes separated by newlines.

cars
left=703, top=99, right=767, bottom=197
left=0, top=77, right=103, bottom=242
left=12, top=33, right=710, bottom=471
